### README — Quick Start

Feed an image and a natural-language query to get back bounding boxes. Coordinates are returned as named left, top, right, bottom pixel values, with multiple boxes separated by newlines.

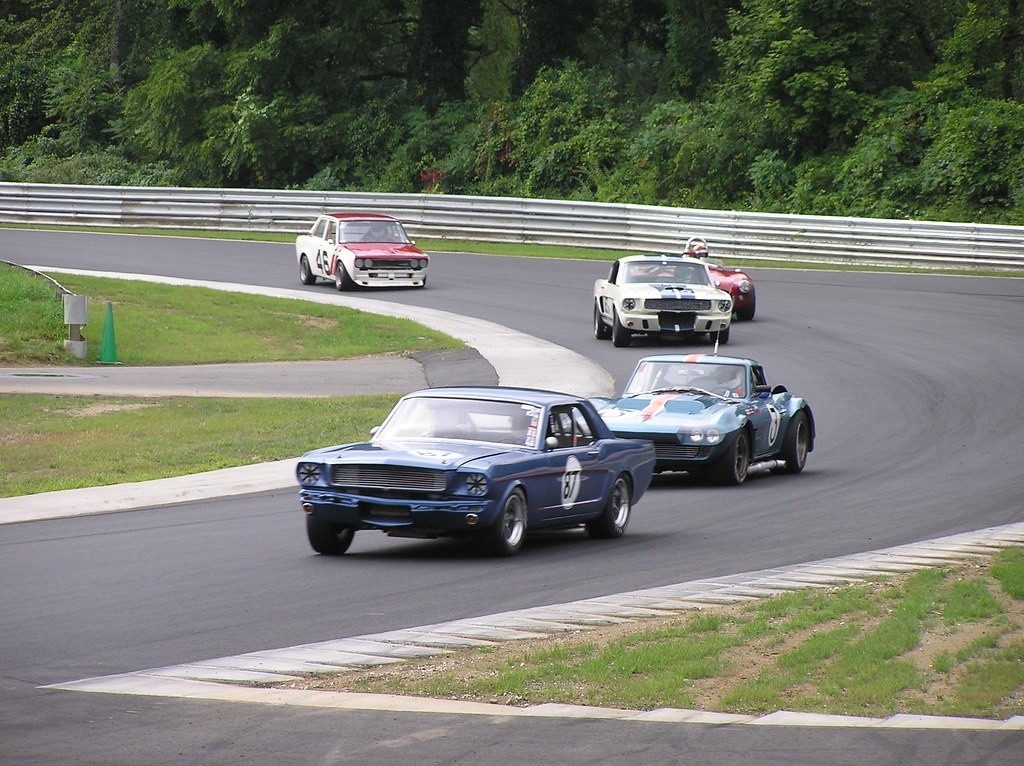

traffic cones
left=95, top=301, right=124, bottom=366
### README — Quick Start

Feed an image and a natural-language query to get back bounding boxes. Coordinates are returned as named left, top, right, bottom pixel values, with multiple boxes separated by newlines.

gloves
left=722, top=390, right=734, bottom=398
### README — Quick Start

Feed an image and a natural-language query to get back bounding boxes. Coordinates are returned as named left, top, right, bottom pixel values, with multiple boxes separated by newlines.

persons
left=362, top=224, right=397, bottom=242
left=715, top=366, right=745, bottom=397
left=673, top=265, right=695, bottom=283
left=507, top=407, right=557, bottom=451
left=683, top=244, right=709, bottom=259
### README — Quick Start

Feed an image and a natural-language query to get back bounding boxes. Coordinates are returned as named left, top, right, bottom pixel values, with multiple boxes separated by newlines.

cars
left=593, top=252, right=735, bottom=348
left=294, top=385, right=658, bottom=556
left=295, top=212, right=431, bottom=292
left=573, top=353, right=816, bottom=486
left=631, top=258, right=756, bottom=322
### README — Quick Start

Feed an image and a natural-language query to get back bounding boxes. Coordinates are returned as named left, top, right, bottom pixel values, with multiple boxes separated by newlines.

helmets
left=693, top=245, right=708, bottom=260
left=675, top=266, right=692, bottom=283
left=369, top=224, right=385, bottom=237
left=715, top=366, right=740, bottom=389
left=509, top=414, right=529, bottom=437
